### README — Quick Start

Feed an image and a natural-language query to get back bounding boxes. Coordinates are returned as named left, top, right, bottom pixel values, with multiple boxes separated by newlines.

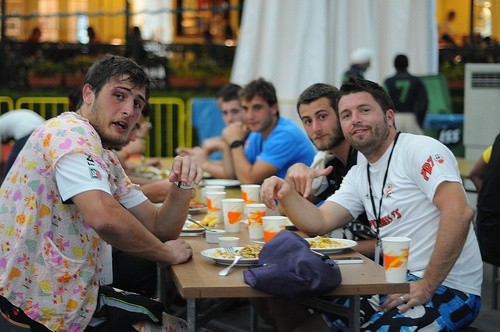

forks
left=223, top=246, right=236, bottom=255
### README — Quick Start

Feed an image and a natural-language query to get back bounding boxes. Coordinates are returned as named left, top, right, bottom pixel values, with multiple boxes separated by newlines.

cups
left=206, top=229, right=225, bottom=242
left=381, top=237, right=411, bottom=283
left=241, top=185, right=261, bottom=205
left=203, top=185, right=227, bottom=223
left=260, top=216, right=287, bottom=243
left=221, top=198, right=245, bottom=231
left=219, top=236, right=239, bottom=248
left=247, top=202, right=267, bottom=239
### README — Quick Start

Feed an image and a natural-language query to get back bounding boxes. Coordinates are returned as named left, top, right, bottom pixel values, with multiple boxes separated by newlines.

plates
left=182, top=220, right=209, bottom=231
left=202, top=179, right=241, bottom=186
left=286, top=217, right=296, bottom=230
left=303, top=237, right=358, bottom=254
left=201, top=247, right=262, bottom=266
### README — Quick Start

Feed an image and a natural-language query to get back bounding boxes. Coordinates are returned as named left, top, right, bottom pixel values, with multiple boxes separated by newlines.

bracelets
left=229, top=140, right=244, bottom=151
left=173, top=180, right=195, bottom=189
left=121, top=147, right=130, bottom=158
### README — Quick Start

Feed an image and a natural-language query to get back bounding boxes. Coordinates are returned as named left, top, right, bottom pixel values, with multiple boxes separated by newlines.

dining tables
left=155, top=187, right=410, bottom=332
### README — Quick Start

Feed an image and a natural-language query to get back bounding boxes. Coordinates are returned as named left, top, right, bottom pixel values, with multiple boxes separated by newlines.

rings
left=400, top=297, right=407, bottom=303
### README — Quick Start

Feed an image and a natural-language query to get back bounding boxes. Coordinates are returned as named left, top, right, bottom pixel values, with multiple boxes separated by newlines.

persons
left=128, top=23, right=145, bottom=47
left=437, top=11, right=500, bottom=66
left=173, top=82, right=249, bottom=179
left=261, top=82, right=484, bottom=332
left=199, top=31, right=229, bottom=68
left=223, top=79, right=321, bottom=185
left=279, top=84, right=383, bottom=265
left=85, top=24, right=100, bottom=45
left=386, top=54, right=429, bottom=126
left=0, top=52, right=214, bottom=332
left=339, top=48, right=373, bottom=85
left=26, top=27, right=43, bottom=41
